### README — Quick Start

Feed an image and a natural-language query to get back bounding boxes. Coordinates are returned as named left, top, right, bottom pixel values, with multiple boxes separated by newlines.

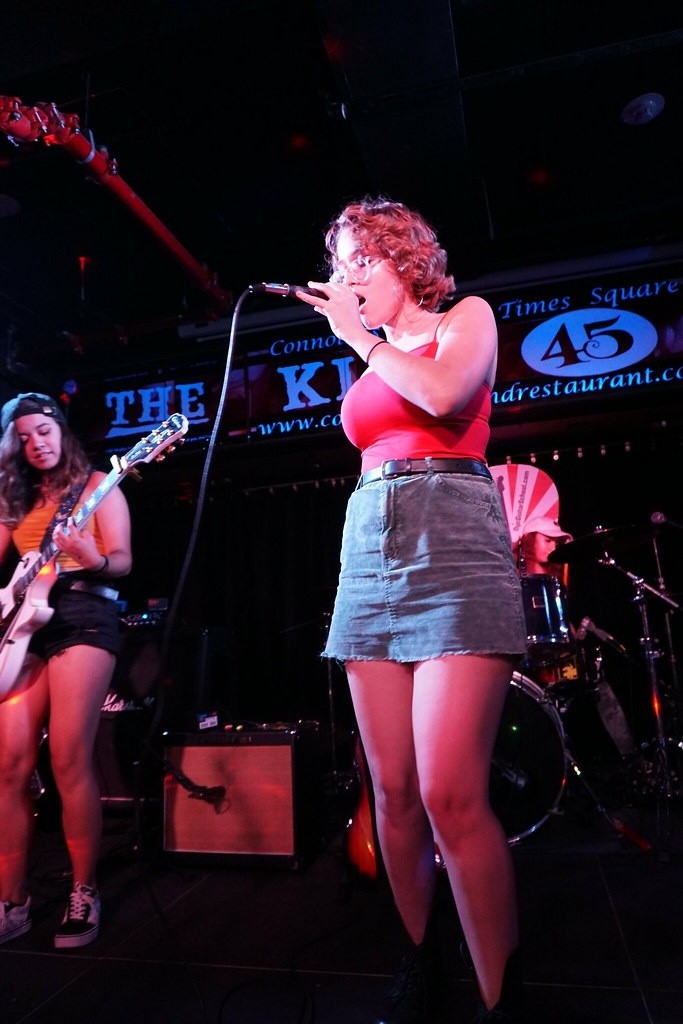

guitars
left=0, top=410, right=192, bottom=702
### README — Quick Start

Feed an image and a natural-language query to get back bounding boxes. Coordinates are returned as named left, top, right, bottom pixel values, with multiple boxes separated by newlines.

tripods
left=597, top=547, right=683, bottom=837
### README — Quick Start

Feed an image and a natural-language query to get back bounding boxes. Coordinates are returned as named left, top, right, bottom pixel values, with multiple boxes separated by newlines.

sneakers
left=0, top=892, right=31, bottom=944
left=53, top=881, right=100, bottom=949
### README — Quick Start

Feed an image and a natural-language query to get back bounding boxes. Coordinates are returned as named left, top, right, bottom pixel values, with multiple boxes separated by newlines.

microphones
left=651, top=511, right=683, bottom=530
left=508, top=772, right=528, bottom=790
left=187, top=787, right=225, bottom=802
left=250, top=281, right=331, bottom=303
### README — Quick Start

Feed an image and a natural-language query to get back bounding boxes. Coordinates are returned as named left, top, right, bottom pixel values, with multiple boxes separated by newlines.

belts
left=358, top=458, right=492, bottom=489
left=51, top=578, right=120, bottom=602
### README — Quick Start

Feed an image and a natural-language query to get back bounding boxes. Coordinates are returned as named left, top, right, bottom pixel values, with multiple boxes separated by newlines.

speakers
left=93, top=623, right=210, bottom=812
left=162, top=723, right=323, bottom=874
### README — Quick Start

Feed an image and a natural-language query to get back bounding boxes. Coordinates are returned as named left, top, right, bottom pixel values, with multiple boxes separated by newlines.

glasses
left=328, top=256, right=391, bottom=286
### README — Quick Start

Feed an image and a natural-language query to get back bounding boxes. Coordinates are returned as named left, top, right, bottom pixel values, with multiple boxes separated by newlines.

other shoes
left=376, top=944, right=445, bottom=1024
left=468, top=1002, right=533, bottom=1024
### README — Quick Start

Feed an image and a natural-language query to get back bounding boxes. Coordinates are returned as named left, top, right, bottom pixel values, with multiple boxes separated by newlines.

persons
left=296, top=205, right=529, bottom=1010
left=510, top=518, right=574, bottom=578
left=1, top=390, right=131, bottom=948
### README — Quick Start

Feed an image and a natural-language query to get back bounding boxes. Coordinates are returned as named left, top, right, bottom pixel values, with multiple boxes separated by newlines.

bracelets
left=365, top=341, right=389, bottom=364
left=98, top=554, right=109, bottom=572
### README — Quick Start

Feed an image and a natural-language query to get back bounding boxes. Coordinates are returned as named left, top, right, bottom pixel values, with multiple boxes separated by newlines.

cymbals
left=277, top=613, right=331, bottom=635
left=546, top=518, right=646, bottom=565
left=615, top=519, right=682, bottom=555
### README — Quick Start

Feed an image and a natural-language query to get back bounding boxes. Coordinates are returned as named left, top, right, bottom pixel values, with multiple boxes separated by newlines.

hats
left=2, top=392, right=67, bottom=433
left=522, top=517, right=573, bottom=544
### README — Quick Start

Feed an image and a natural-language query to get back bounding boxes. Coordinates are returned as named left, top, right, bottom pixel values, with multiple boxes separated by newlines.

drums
left=484, top=668, right=572, bottom=850
left=516, top=573, right=574, bottom=655
